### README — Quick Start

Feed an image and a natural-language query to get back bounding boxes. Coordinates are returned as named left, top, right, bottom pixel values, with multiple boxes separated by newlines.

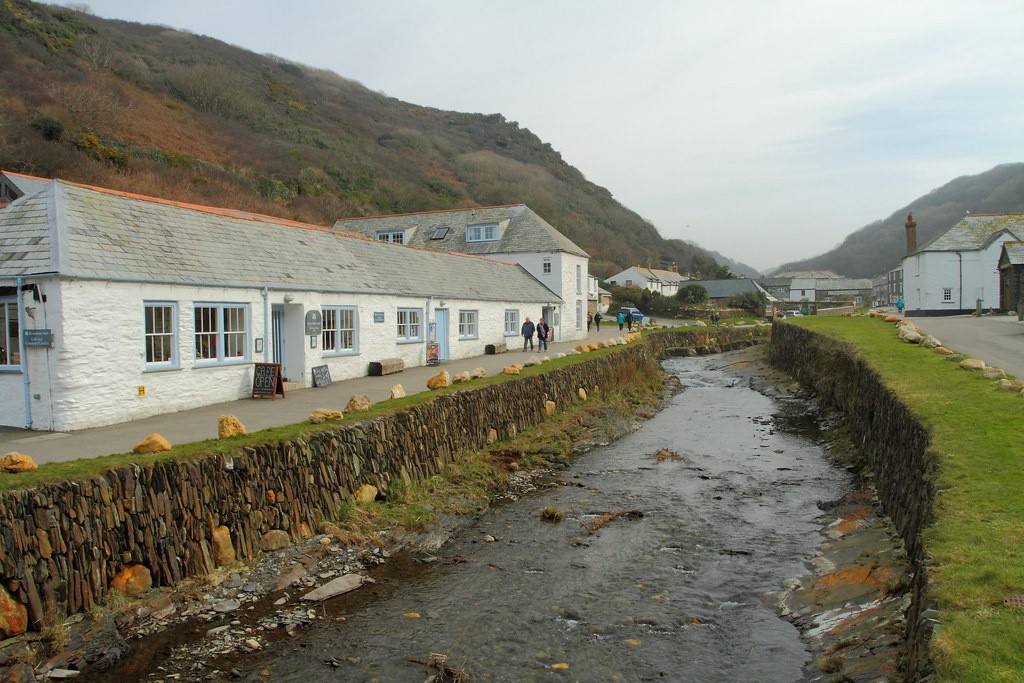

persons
left=522, top=317, right=535, bottom=352
left=617, top=312, right=625, bottom=331
left=710, top=313, right=720, bottom=326
left=896, top=298, right=904, bottom=314
left=588, top=312, right=593, bottom=331
left=625, top=311, right=633, bottom=333
left=537, top=318, right=549, bottom=353
left=594, top=312, right=602, bottom=332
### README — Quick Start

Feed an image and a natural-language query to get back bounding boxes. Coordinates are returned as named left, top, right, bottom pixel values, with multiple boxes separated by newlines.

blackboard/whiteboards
left=312, top=365, right=333, bottom=388
left=253, top=363, right=284, bottom=396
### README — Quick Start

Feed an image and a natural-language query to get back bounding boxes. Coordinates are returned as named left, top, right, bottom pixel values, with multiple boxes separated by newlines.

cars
left=783, top=309, right=804, bottom=317
left=616, top=307, right=644, bottom=323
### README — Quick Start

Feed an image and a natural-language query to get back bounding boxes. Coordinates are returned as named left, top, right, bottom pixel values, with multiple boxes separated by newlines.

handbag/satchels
left=599, top=316, right=602, bottom=318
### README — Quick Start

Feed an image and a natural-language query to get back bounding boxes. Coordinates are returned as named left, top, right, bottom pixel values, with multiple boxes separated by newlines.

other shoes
left=545, top=350, right=547, bottom=352
left=531, top=349, right=533, bottom=351
left=538, top=350, right=541, bottom=352
left=523, top=350, right=527, bottom=352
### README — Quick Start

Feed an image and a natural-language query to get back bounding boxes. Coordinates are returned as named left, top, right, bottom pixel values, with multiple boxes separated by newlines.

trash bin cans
left=810, top=305, right=817, bottom=315
